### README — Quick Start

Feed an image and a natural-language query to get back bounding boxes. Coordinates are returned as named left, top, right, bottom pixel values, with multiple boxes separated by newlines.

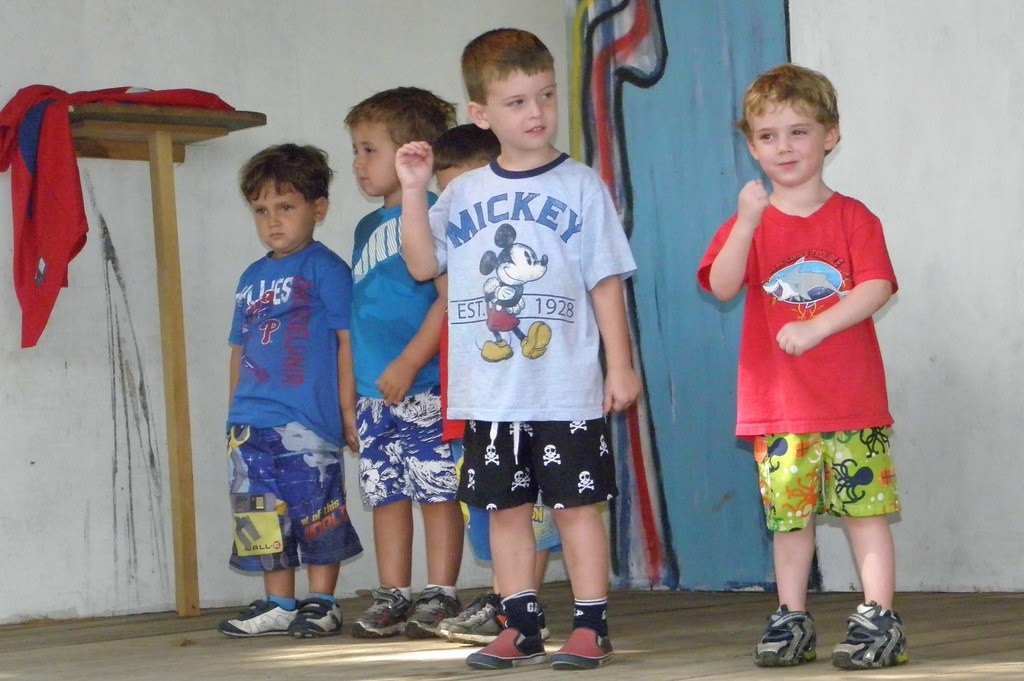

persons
left=395, top=26, right=643, bottom=673
left=216, top=144, right=360, bottom=640
left=695, top=65, right=908, bottom=671
left=345, top=86, right=561, bottom=645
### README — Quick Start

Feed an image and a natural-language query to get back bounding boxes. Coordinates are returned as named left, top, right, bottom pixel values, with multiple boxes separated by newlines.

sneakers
left=352, top=585, right=415, bottom=637
left=550, top=626, right=615, bottom=669
left=405, top=586, right=464, bottom=638
left=832, top=600, right=908, bottom=670
left=435, top=591, right=508, bottom=644
left=466, top=628, right=547, bottom=670
left=288, top=598, right=342, bottom=638
left=217, top=599, right=300, bottom=637
left=752, top=603, right=816, bottom=666
